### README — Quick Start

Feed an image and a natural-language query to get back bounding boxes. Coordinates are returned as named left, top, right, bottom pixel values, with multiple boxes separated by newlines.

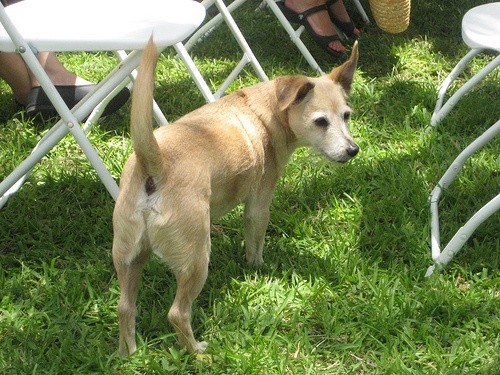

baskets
left=369, top=0, right=410, bottom=34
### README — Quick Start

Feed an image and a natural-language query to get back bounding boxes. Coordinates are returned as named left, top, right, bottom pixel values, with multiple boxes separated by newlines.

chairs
left=422, top=0, right=500, bottom=280
left=1, top=0, right=371, bottom=213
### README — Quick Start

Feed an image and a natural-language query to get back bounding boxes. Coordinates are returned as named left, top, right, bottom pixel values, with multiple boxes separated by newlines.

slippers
left=326, top=0, right=360, bottom=39
left=276, top=0, right=345, bottom=57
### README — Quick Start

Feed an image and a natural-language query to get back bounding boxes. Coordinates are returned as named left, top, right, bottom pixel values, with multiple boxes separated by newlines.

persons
left=276, top=0, right=361, bottom=57
left=0, top=51, right=131, bottom=123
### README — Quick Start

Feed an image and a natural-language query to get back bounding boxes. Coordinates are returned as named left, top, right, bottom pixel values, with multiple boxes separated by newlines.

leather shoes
left=26, top=83, right=130, bottom=122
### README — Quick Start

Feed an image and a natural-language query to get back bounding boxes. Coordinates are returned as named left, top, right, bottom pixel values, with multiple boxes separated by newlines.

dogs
left=111, top=31, right=360, bottom=355
